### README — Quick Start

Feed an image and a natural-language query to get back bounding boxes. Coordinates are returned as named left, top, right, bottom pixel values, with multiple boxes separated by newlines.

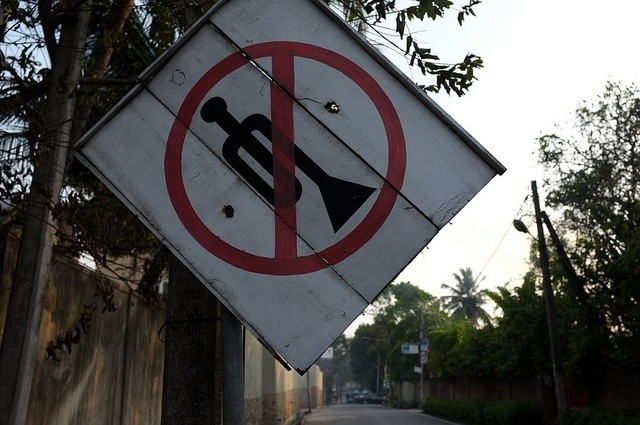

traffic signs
left=70, top=0, right=508, bottom=376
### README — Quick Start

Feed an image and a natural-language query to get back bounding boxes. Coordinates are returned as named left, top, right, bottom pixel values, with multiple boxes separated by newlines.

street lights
left=514, top=181, right=569, bottom=425
